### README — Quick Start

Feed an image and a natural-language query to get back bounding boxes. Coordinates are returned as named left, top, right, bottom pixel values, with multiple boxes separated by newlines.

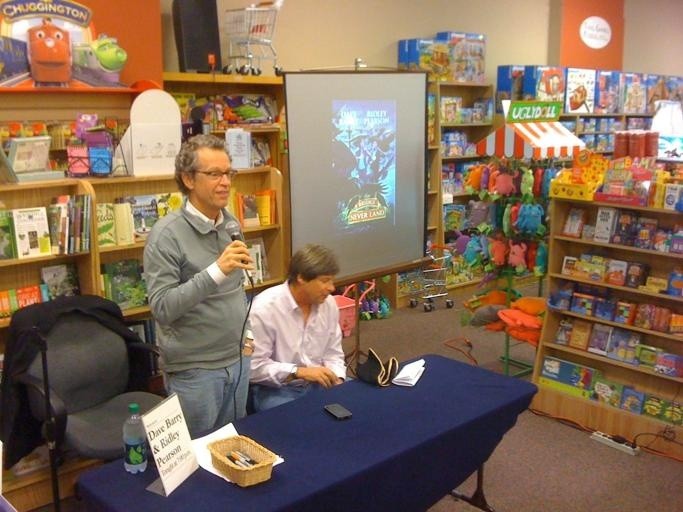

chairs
left=12, top=295, right=165, bottom=510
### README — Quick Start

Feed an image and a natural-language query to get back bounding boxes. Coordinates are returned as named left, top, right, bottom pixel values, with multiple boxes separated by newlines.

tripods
left=344, top=283, right=371, bottom=376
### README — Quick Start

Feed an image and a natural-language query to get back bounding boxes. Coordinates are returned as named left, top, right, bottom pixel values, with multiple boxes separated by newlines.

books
left=226, top=188, right=276, bottom=229
left=0, top=191, right=188, bottom=377
left=239, top=236, right=270, bottom=286
left=15, top=170, right=65, bottom=182
left=8, top=135, right=52, bottom=174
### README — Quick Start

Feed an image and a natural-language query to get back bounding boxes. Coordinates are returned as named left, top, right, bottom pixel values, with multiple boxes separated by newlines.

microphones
left=225, top=220, right=253, bottom=282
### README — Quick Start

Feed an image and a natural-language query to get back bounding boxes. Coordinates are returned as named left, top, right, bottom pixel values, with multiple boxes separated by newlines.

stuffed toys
left=462, top=286, right=548, bottom=347
left=450, top=156, right=565, bottom=277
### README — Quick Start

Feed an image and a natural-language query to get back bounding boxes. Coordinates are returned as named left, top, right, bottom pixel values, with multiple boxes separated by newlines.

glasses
left=191, top=168, right=238, bottom=182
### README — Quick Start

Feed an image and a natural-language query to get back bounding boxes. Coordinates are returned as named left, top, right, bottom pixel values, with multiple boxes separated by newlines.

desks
left=77, top=353, right=539, bottom=509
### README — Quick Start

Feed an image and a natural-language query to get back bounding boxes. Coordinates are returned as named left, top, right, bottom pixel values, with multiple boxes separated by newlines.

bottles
left=124, top=403, right=148, bottom=474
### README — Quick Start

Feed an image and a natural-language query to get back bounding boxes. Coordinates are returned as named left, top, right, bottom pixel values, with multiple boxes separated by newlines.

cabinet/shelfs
left=529, top=161, right=683, bottom=458
left=397, top=78, right=442, bottom=310
left=0, top=71, right=284, bottom=330
left=439, top=78, right=497, bottom=300
left=557, top=113, right=653, bottom=156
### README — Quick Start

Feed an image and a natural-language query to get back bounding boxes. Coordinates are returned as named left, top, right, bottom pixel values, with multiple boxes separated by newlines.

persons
left=248, top=245, right=348, bottom=414
left=143, top=134, right=250, bottom=442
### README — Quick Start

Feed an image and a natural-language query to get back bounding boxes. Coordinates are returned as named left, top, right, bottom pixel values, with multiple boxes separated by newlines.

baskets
left=207, top=434, right=279, bottom=488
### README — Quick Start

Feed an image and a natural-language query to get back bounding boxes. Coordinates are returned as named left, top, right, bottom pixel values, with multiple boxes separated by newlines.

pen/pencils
left=237, top=451, right=259, bottom=465
left=231, top=450, right=252, bottom=468
left=227, top=456, right=248, bottom=468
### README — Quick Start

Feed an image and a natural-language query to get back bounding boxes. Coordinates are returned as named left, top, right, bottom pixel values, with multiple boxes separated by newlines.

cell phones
left=324, top=403, right=352, bottom=420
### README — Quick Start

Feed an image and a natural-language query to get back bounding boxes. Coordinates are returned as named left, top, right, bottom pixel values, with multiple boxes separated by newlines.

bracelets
left=244, top=343, right=255, bottom=352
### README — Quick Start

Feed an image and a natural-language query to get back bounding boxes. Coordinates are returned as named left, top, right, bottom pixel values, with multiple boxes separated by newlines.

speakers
left=171, top=0, right=222, bottom=73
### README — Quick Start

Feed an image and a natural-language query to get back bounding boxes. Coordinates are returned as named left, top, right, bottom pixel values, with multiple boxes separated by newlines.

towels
left=392, top=359, right=425, bottom=387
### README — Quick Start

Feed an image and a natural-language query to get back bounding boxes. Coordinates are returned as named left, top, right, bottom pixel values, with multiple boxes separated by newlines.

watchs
left=291, top=367, right=298, bottom=379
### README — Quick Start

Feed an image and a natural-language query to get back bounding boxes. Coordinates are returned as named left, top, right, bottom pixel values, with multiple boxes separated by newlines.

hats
left=356, top=348, right=400, bottom=386
